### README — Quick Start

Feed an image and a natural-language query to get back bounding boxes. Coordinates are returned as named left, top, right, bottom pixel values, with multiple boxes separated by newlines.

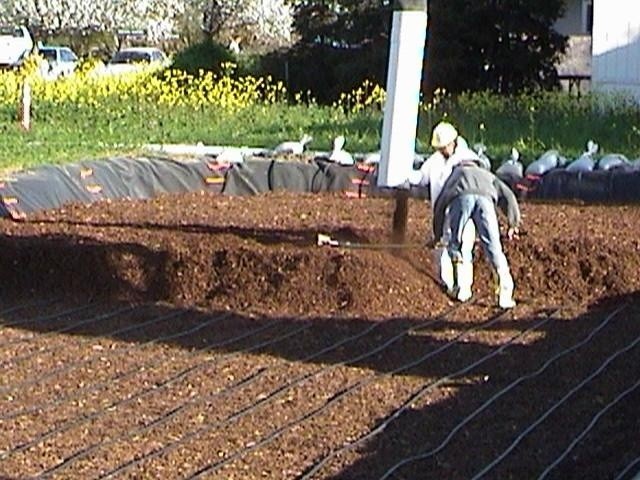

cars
left=102, top=47, right=168, bottom=79
left=0, top=25, right=78, bottom=85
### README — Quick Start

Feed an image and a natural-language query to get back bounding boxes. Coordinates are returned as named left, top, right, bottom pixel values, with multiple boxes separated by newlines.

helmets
left=431, top=122, right=457, bottom=148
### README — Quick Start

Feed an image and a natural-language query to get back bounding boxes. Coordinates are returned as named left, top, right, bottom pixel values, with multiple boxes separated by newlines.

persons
left=410, top=122, right=487, bottom=288
left=425, top=157, right=523, bottom=310
